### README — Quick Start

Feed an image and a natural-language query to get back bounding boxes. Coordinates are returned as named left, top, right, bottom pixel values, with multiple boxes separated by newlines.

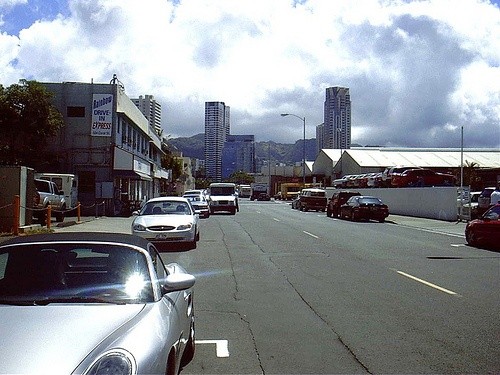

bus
left=207, top=182, right=237, bottom=215
left=238, top=184, right=252, bottom=198
left=280, top=182, right=323, bottom=201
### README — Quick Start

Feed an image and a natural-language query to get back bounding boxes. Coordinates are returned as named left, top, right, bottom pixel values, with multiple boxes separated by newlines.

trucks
left=250, top=182, right=268, bottom=201
left=34, top=173, right=79, bottom=212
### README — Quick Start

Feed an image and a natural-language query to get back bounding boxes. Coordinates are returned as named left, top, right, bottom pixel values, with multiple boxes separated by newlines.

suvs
left=34, top=178, right=67, bottom=227
left=327, top=191, right=362, bottom=219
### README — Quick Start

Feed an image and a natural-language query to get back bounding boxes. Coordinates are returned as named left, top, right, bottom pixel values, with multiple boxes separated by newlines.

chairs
left=177, top=206, right=184, bottom=212
left=107, top=253, right=136, bottom=275
left=29, top=263, right=67, bottom=292
left=153, top=207, right=161, bottom=213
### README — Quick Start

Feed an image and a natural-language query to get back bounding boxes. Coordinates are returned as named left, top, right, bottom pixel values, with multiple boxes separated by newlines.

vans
left=298, top=188, right=327, bottom=212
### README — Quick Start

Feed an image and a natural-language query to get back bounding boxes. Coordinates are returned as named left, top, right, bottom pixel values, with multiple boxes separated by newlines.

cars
left=456, top=192, right=500, bottom=222
left=0, top=231, right=198, bottom=375
left=464, top=204, right=500, bottom=253
left=339, top=196, right=389, bottom=223
left=181, top=189, right=211, bottom=219
left=131, top=195, right=200, bottom=251
left=292, top=197, right=301, bottom=209
left=331, top=166, right=457, bottom=189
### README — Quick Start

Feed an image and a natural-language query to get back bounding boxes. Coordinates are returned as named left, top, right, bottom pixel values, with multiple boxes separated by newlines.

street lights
left=280, top=113, right=306, bottom=184
